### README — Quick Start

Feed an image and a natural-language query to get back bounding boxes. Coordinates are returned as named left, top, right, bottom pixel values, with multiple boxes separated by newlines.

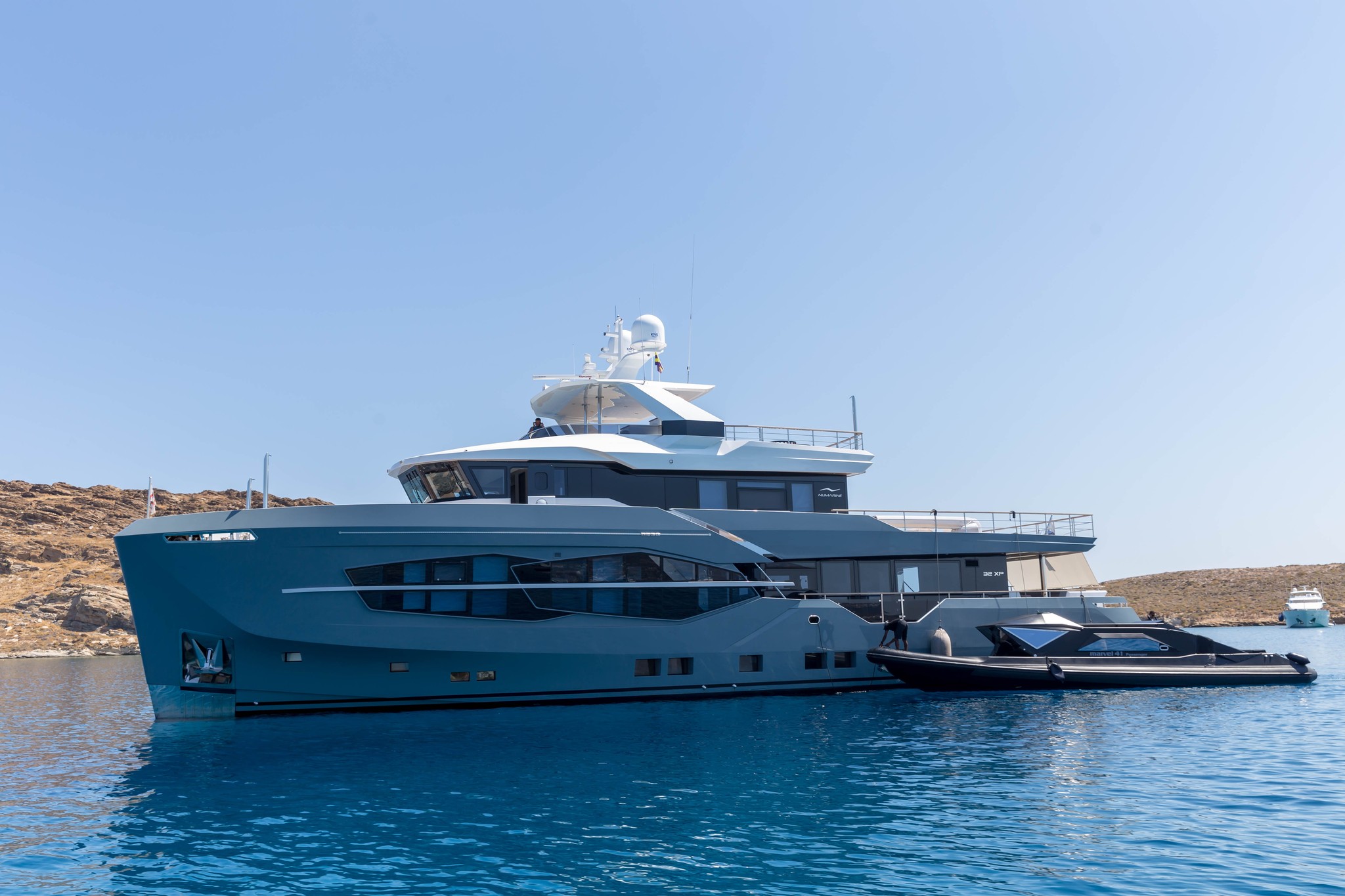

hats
left=883, top=621, right=889, bottom=630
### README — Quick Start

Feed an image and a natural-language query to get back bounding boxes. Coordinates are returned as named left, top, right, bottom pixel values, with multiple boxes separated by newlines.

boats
left=864, top=610, right=1318, bottom=693
left=112, top=305, right=1145, bottom=723
left=1328, top=621, right=1336, bottom=626
left=1282, top=577, right=1330, bottom=629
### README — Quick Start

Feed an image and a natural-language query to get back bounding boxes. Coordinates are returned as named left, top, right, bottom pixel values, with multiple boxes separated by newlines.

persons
left=879, top=618, right=908, bottom=651
left=527, top=418, right=545, bottom=433
left=1144, top=611, right=1155, bottom=621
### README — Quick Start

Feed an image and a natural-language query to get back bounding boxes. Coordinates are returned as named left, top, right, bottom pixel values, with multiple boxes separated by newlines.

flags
left=150, top=480, right=156, bottom=515
left=655, top=352, right=664, bottom=375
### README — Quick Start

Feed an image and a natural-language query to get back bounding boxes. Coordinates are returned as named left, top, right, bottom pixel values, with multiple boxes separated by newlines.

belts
left=897, top=619, right=904, bottom=624
